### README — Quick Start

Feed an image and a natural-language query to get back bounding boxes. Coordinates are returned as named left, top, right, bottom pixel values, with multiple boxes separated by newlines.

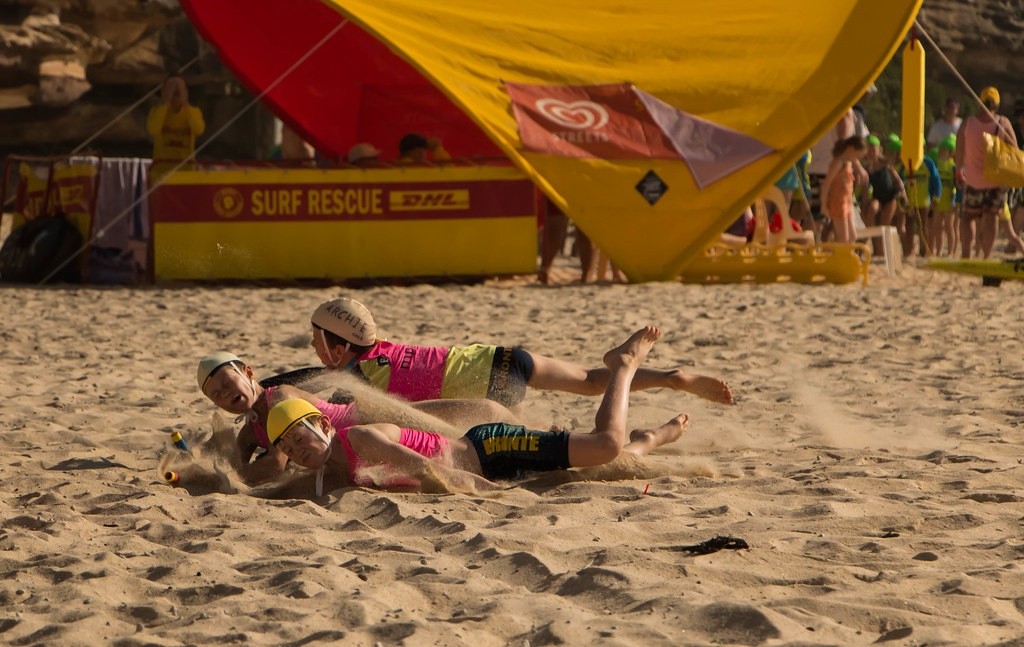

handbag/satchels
left=982, top=116, right=1024, bottom=189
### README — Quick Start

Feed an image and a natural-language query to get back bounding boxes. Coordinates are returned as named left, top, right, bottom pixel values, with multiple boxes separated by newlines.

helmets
left=266, top=398, right=323, bottom=448
left=311, top=298, right=377, bottom=347
left=197, top=351, right=245, bottom=391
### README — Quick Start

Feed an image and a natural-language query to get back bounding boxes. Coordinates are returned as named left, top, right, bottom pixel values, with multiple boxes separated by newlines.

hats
left=884, top=134, right=901, bottom=151
left=868, top=135, right=880, bottom=145
left=400, top=131, right=436, bottom=153
left=939, top=133, right=957, bottom=151
left=980, top=87, right=1000, bottom=105
left=347, top=142, right=378, bottom=159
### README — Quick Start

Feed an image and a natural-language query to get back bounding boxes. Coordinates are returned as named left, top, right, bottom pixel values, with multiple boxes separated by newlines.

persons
left=537, top=197, right=593, bottom=283
left=196, top=298, right=733, bottom=501
left=399, top=133, right=435, bottom=166
left=725, top=86, right=1024, bottom=262
left=349, top=143, right=380, bottom=168
left=145, top=73, right=204, bottom=173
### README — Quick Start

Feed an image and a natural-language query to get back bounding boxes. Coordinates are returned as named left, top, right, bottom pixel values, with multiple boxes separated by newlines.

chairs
left=851, top=208, right=903, bottom=276
left=749, top=188, right=816, bottom=251
